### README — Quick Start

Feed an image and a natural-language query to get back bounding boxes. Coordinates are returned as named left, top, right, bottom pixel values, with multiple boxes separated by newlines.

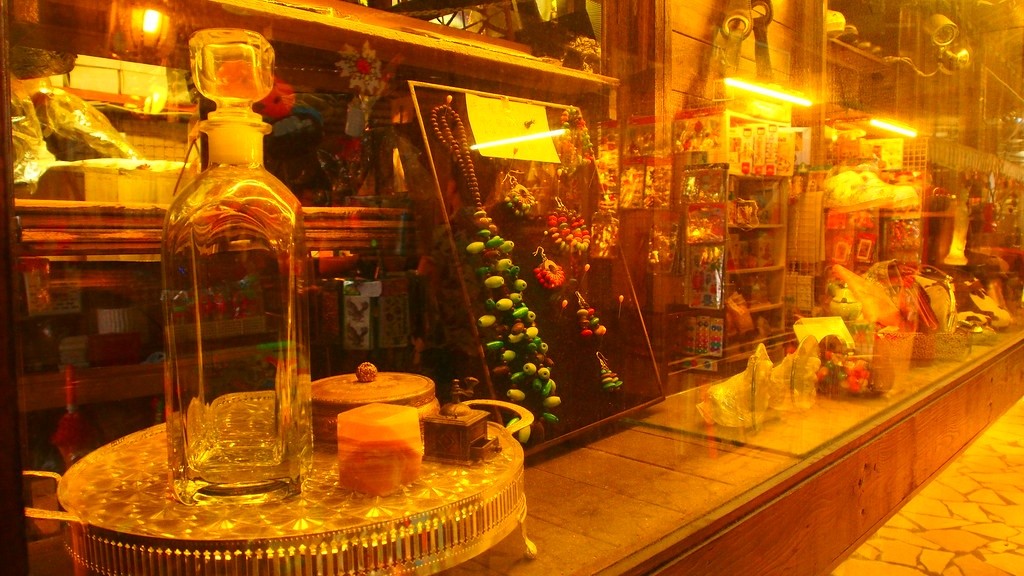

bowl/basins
left=968, top=248, right=1017, bottom=270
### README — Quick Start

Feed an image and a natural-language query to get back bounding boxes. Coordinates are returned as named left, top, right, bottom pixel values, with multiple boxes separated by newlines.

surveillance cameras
left=924, top=13, right=960, bottom=47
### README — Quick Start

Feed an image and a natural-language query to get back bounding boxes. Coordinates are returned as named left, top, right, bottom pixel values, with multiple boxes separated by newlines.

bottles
left=163, top=29, right=315, bottom=502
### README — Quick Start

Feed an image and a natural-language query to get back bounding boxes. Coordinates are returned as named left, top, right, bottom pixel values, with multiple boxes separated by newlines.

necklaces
left=434, top=107, right=561, bottom=444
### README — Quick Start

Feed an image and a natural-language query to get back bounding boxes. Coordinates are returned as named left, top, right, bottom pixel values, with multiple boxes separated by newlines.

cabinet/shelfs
left=672, top=108, right=795, bottom=362
left=9, top=0, right=625, bottom=422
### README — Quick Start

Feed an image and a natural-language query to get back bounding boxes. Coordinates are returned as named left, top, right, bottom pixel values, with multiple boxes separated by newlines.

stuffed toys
left=253, top=78, right=323, bottom=185
